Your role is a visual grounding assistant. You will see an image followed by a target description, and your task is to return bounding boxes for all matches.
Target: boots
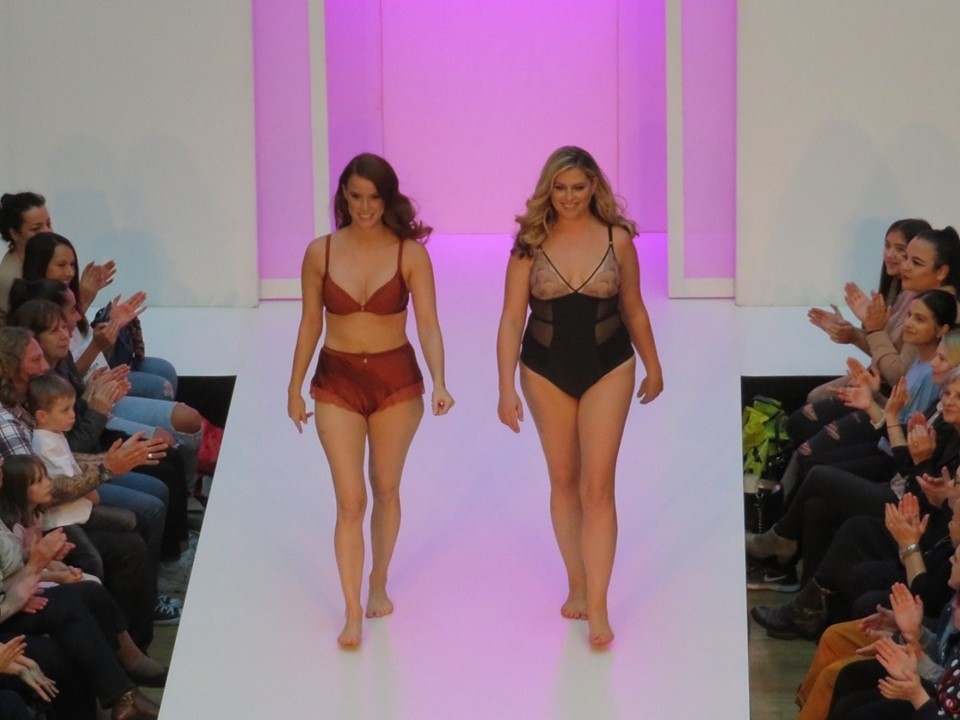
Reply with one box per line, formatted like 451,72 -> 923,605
750,578 -> 837,642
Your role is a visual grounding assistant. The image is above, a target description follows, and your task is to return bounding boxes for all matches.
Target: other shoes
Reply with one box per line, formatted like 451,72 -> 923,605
157,593 -> 183,608
153,600 -> 183,625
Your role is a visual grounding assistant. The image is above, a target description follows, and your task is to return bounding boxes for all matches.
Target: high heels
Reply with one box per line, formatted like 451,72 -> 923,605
745,524 -> 796,566
112,688 -> 159,719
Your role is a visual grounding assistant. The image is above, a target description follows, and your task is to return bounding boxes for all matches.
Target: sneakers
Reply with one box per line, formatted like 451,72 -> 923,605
164,556 -> 194,581
746,565 -> 801,592
180,545 -> 196,564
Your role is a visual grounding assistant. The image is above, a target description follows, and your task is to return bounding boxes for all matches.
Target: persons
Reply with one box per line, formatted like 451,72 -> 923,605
745,218 -> 960,719
496,147 -> 664,646
0,190 -> 211,720
287,152 -> 454,648
